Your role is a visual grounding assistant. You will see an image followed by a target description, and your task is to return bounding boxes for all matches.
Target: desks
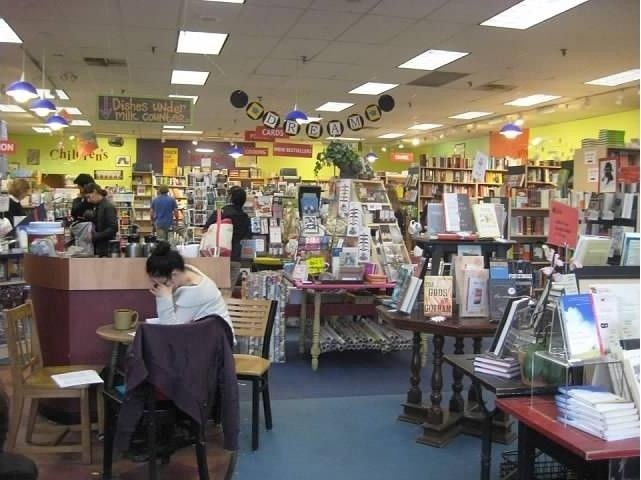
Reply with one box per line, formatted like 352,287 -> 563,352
284,276 -> 429,372
441,349 -> 570,480
494,397 -> 639,479
96,319 -> 146,389
19,250 -> 232,427
368,302 -> 519,450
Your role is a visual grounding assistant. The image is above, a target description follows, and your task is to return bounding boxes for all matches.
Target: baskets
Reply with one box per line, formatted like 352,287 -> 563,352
500,448 -> 584,480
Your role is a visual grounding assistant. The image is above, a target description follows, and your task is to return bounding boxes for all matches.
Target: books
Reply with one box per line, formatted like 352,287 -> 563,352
0,178 -> 52,225
52,195 -> 133,249
401,279 -> 423,315
153,177 -> 176,238
411,154 -> 559,321
396,275 -> 418,313
132,166 -> 151,240
50,368 -> 105,389
246,178 -> 409,311
176,166 -> 264,244
568,130 -> 638,232
476,232 -> 640,441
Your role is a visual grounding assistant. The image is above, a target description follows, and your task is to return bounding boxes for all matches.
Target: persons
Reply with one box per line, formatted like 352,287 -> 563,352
69,173 -> 98,221
150,184 -> 178,240
73,182 -> 120,259
82,240 -> 241,410
204,185 -> 253,290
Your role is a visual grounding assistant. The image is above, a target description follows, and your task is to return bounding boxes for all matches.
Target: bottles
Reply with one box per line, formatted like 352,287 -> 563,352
144,235 -> 160,257
127,234 -> 144,257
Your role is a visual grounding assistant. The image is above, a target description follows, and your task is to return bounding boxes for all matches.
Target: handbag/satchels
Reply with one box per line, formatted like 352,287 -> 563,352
200,208 -> 234,257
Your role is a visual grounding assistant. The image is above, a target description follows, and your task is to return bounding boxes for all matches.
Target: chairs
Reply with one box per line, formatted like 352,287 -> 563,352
0,297 -> 105,468
220,299 -> 278,453
99,310 -> 213,479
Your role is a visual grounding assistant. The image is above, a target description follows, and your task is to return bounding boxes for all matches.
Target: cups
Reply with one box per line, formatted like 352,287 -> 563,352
113,309 -> 139,329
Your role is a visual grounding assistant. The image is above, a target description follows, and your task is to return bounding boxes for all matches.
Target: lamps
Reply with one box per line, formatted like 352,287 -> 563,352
1,43 -> 41,106
43,92 -> 70,130
27,52 -> 58,117
495,122 -> 523,139
282,102 -> 309,125
